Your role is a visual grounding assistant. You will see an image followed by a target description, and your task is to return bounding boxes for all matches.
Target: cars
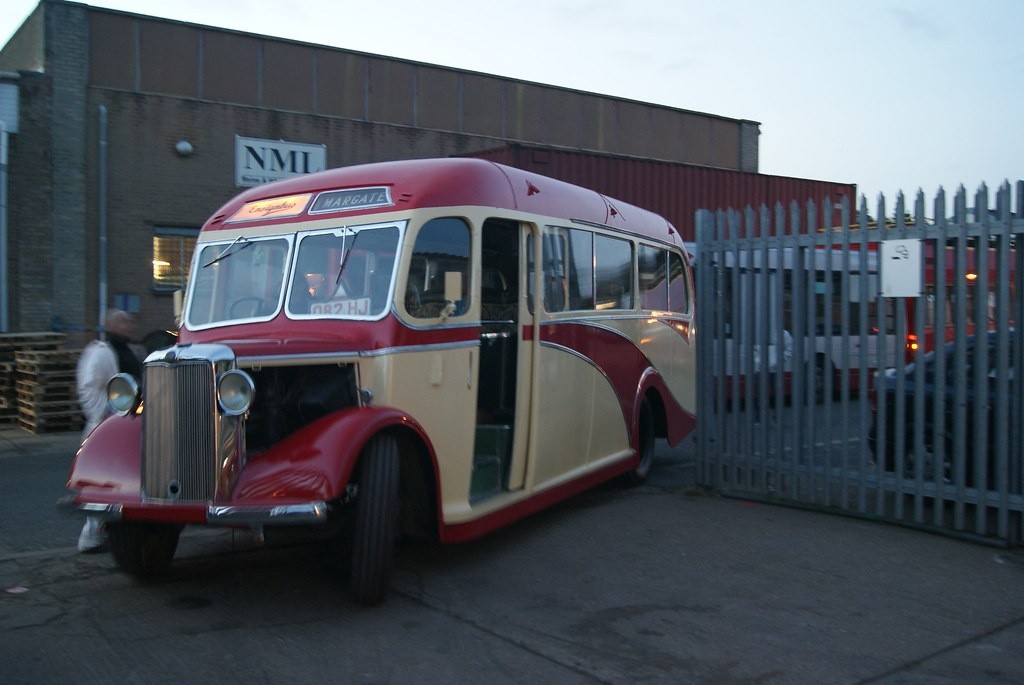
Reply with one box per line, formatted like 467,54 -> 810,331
867,326 -> 1023,517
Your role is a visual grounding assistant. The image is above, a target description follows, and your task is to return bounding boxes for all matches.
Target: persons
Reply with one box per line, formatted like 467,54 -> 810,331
76,309 -> 148,553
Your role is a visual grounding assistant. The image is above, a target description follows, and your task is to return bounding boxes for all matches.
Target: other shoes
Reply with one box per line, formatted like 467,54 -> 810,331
83,537 -> 114,554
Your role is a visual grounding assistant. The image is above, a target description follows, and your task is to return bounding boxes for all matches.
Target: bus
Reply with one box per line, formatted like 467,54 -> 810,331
673,241 -> 919,413
813,219 -> 1021,364
67,152 -> 697,611
